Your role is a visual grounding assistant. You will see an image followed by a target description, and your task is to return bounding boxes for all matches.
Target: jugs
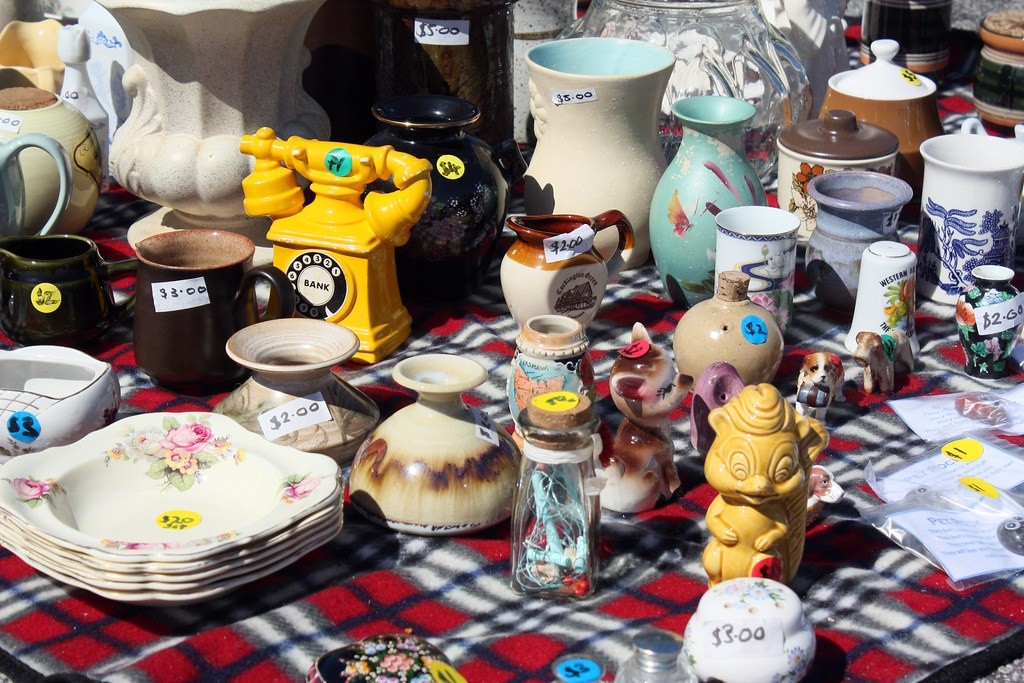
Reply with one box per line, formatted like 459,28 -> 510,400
134,230 -> 295,395
0,234 -> 137,351
500,210 -> 635,329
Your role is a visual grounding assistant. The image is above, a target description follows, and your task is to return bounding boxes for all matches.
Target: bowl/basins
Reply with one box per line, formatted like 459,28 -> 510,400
776,109 -> 899,239
0,346 -> 121,455
815,39 -> 945,226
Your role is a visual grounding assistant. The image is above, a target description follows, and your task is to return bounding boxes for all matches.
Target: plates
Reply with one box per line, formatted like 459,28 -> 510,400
0,412 -> 345,607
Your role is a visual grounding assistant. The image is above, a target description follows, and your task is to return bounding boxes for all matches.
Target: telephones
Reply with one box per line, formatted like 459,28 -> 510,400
238,124 -> 434,366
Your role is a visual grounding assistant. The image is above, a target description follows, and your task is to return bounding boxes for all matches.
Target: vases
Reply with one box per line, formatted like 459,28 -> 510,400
1,0 -> 848,307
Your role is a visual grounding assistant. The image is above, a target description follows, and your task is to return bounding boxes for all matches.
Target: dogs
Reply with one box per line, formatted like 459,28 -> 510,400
806,464 -> 846,510
853,331 -> 916,398
794,352 -> 847,427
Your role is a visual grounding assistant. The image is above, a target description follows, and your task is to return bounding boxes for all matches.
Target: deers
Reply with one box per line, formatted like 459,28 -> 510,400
595,321 -> 694,513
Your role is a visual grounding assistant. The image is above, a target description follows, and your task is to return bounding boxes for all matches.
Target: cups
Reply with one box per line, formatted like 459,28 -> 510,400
861,0 -> 953,79
916,134 -> 1024,305
0,133 -> 72,235
714,206 -> 800,334
845,241 -> 920,354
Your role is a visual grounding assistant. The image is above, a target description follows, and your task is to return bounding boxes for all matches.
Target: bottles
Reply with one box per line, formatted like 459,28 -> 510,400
972,9 -> 1024,126
508,391 -> 600,599
213,317 -> 379,467
524,38 -> 676,269
955,265 -> 1024,379
507,315 -> 596,453
361,94 -> 528,304
673,271 -> 784,386
613,630 -> 698,683
346,354 -> 522,534
649,96 -> 769,308
54,23 -> 112,193
